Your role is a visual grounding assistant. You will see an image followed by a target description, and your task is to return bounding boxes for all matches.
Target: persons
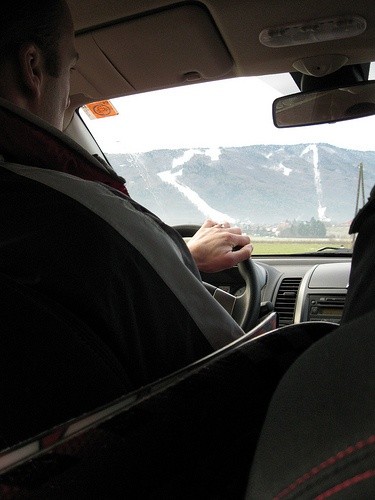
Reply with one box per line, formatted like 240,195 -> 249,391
0,0 -> 255,500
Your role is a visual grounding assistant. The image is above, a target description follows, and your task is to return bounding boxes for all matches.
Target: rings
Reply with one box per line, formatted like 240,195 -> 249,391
218,223 -> 225,228
